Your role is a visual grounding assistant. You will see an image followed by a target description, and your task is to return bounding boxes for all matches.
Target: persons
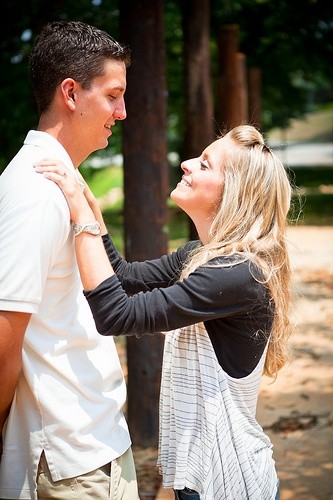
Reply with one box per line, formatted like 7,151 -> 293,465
0,21 -> 139,500
32,125 -> 304,500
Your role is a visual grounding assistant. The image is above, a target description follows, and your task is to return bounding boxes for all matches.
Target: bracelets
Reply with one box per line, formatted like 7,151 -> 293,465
72,221 -> 101,237
